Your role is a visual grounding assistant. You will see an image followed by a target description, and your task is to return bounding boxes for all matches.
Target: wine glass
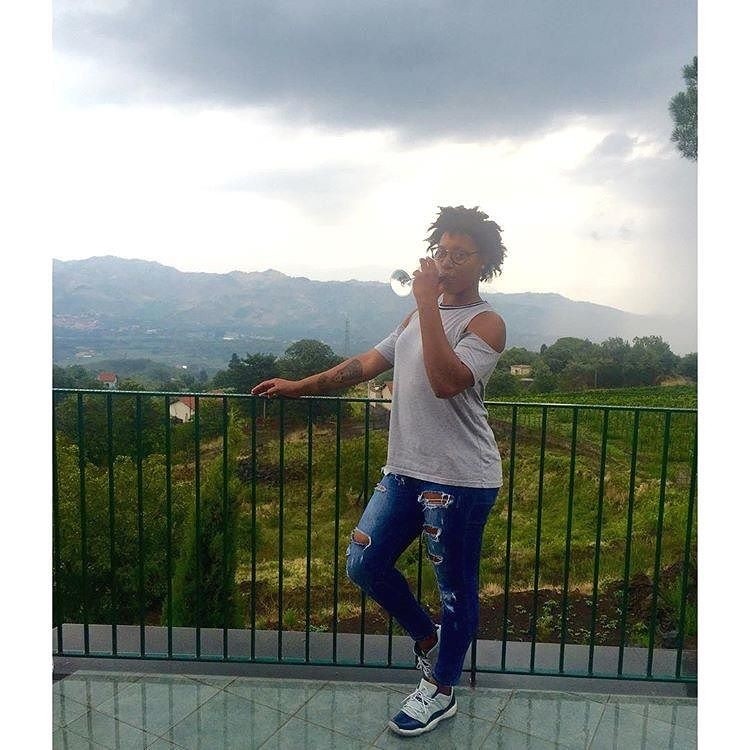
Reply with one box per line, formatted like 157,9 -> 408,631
390,260 -> 452,297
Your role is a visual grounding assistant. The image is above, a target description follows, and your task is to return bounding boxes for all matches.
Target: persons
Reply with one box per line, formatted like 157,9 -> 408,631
251,205 -> 506,736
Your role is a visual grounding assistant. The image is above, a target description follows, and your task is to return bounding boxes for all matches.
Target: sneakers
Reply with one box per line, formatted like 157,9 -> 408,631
387,679 -> 457,737
413,623 -> 442,678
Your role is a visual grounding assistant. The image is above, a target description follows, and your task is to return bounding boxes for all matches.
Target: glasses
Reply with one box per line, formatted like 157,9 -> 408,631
430,246 -> 481,266
